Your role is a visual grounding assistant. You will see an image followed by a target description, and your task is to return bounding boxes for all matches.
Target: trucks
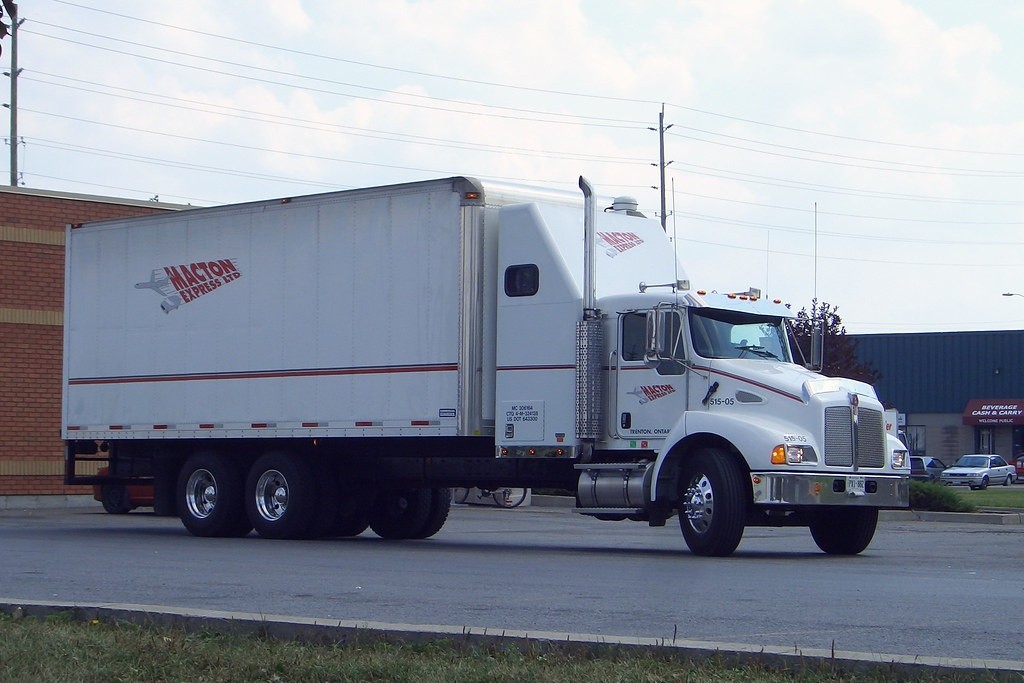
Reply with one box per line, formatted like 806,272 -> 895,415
882,407 -> 912,453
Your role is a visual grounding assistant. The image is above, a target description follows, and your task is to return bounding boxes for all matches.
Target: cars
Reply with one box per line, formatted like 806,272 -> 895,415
1008,453 -> 1024,484
939,454 -> 1016,491
910,455 -> 951,482
90,463 -> 156,515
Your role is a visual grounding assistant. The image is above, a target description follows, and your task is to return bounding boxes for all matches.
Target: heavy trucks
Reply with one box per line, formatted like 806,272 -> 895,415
61,173 -> 914,557
